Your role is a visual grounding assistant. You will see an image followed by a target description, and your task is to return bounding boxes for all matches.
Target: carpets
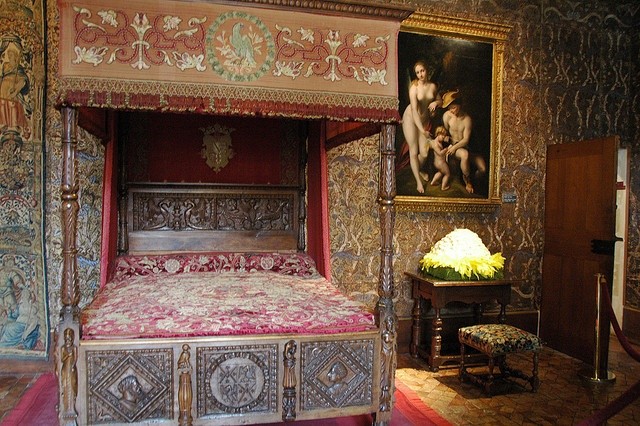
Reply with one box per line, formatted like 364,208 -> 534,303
1,370 -> 455,426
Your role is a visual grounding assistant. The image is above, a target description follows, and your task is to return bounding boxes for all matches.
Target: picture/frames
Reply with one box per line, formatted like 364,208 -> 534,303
394,10 -> 516,212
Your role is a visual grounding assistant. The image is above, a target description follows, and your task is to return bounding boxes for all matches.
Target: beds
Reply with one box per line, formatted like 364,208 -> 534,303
61,1 -> 419,423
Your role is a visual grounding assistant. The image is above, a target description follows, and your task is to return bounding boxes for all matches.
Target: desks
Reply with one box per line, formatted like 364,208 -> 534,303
403,269 -> 525,371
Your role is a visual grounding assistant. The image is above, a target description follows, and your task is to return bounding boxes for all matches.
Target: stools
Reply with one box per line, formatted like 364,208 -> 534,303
459,323 -> 546,395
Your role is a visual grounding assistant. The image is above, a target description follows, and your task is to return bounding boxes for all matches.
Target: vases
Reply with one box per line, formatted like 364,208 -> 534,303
421,265 -> 503,283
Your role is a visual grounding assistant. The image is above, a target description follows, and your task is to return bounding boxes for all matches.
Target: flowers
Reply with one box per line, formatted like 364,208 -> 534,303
419,228 -> 507,281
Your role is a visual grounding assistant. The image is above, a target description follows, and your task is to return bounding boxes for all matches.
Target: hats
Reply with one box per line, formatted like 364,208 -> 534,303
441,89 -> 463,109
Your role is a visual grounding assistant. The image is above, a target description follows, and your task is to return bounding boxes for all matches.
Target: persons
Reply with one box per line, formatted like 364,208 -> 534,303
442,91 -> 487,193
431,125 -> 451,190
402,62 -> 443,193
